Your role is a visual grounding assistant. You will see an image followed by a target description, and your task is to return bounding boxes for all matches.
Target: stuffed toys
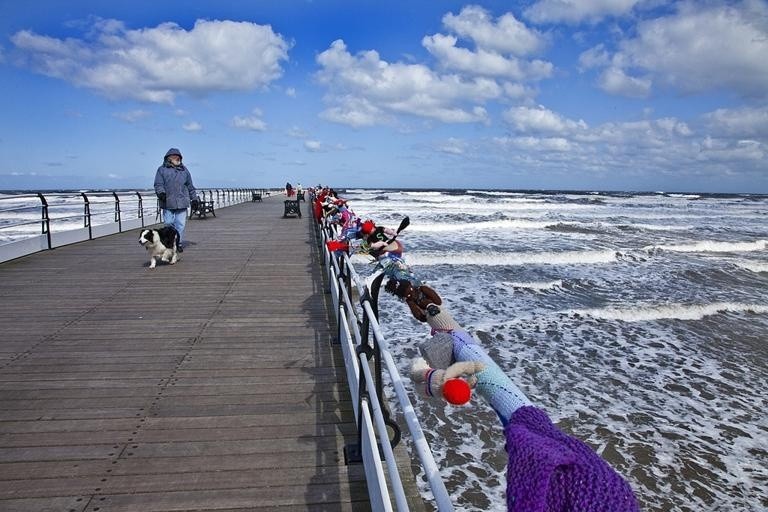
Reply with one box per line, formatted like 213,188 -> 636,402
307,185 -> 483,404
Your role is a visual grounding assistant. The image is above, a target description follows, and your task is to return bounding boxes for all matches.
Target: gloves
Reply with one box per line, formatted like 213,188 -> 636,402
191,200 -> 199,210
158,192 -> 166,202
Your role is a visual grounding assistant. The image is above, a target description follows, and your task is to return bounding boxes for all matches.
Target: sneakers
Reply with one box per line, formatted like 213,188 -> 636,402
176,245 -> 183,252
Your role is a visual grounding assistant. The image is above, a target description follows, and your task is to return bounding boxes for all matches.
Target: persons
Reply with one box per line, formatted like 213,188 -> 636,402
296,183 -> 302,195
154,148 -> 198,253
286,182 -> 292,197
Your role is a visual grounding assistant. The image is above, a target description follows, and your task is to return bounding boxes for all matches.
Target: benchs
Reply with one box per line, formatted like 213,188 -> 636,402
188,196 -> 217,219
251,192 -> 263,202
283,190 -> 306,218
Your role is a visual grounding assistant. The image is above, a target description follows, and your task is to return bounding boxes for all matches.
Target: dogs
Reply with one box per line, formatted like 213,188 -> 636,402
138,226 -> 183,270
